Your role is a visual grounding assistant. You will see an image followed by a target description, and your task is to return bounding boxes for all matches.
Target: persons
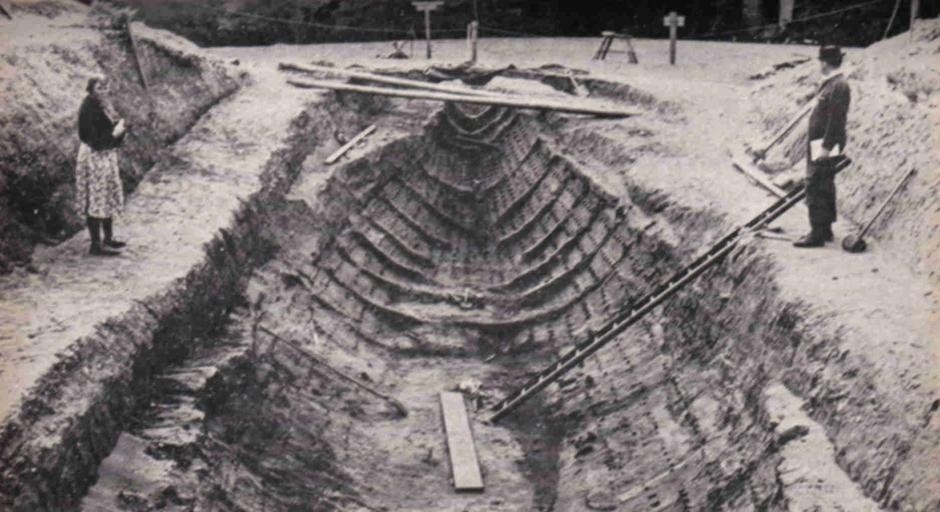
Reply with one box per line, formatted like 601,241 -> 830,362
792,44 -> 851,248
465,13 -> 479,65
74,73 -> 126,255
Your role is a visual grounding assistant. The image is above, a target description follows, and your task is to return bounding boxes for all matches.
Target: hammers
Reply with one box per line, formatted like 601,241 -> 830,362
842,166 -> 914,253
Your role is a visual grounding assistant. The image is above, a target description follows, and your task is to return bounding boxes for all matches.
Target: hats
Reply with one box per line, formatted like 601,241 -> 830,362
818,45 -> 846,64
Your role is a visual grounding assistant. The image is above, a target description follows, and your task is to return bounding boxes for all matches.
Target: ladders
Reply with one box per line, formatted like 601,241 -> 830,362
481,152 -> 853,425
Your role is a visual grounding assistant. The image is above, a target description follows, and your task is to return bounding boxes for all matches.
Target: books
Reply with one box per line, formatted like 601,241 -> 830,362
810,137 -> 839,161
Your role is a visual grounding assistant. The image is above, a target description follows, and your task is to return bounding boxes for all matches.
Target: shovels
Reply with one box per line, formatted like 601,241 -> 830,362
259,325 -> 407,417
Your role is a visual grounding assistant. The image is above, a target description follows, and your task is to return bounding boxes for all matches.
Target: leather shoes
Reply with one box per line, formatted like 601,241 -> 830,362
793,231 -> 825,248
104,238 -> 126,248
90,243 -> 121,255
